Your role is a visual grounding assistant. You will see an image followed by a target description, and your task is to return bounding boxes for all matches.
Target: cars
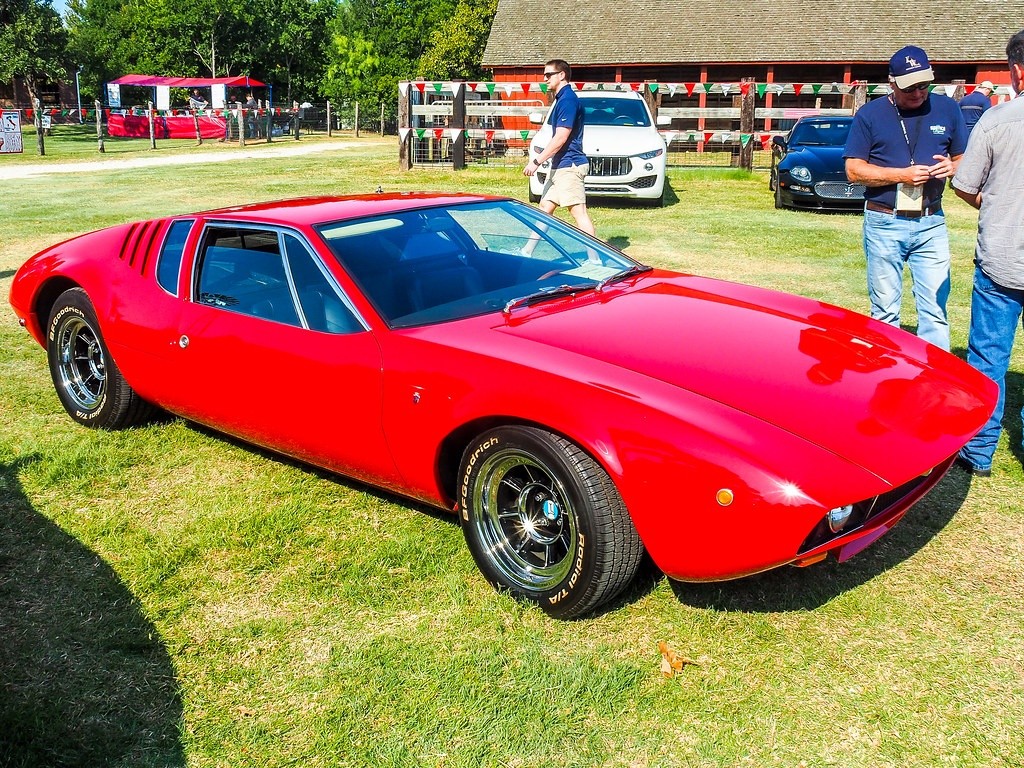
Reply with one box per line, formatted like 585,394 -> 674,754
8,188 -> 1000,620
771,115 -> 874,211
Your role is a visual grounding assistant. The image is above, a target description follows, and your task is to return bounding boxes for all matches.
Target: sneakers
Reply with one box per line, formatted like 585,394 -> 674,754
580,258 -> 603,266
500,247 -> 523,256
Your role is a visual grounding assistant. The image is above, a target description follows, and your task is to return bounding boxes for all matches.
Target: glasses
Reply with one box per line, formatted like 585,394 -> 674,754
544,71 -> 560,78
895,82 -> 930,93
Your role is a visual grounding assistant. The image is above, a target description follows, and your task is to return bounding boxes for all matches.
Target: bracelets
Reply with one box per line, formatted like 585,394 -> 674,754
533,159 -> 540,166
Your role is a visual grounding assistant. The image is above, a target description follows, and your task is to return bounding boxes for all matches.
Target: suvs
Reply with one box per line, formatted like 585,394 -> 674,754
528,89 -> 672,205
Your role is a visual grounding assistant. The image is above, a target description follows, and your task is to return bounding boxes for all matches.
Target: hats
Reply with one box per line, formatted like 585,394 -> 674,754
889,45 -> 934,90
978,81 -> 995,92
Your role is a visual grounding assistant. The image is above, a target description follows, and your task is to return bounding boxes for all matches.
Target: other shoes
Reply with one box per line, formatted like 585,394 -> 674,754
956,455 -> 991,478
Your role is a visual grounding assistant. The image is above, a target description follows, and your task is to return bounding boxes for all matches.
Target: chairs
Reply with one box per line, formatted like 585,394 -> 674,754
397,264 -> 486,315
799,126 -> 821,142
248,288 -> 353,332
608,104 -> 638,123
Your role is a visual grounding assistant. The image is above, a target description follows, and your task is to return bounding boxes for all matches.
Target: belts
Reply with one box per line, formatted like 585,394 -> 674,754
866,200 -> 941,217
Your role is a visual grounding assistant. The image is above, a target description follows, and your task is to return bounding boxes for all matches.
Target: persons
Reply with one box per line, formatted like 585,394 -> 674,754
229,96 -> 236,104
958,80 -> 995,134
841,45 -> 969,353
950,28 -> 1024,478
245,94 -> 258,139
498,60 -> 602,266
186,88 -> 208,108
